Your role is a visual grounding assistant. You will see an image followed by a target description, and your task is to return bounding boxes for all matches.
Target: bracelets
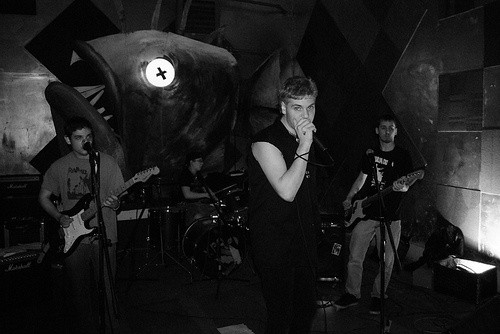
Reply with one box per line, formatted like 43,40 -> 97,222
294,152 -> 309,161
114,200 -> 120,210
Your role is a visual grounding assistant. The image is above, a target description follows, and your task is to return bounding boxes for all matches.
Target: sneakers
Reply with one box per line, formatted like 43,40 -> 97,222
368,297 -> 382,315
334,293 -> 360,309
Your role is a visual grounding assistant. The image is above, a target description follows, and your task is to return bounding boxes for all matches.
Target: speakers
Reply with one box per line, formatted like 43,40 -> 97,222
0,197 -> 60,250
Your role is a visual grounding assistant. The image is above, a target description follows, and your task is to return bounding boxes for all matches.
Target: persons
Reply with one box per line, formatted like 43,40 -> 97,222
246,77 -> 322,334
39,116 -> 128,334
179,152 -> 218,222
333,114 -> 414,314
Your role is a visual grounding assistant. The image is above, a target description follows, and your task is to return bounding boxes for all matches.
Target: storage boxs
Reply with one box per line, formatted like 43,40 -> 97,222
432,256 -> 498,305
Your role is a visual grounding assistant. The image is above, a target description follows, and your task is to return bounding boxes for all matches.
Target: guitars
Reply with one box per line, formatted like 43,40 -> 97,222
47,165 -> 160,257
344,169 -> 425,226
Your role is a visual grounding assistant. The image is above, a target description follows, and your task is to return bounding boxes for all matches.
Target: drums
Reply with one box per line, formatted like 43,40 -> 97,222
224,187 -> 248,218
147,205 -> 186,251
182,215 -> 241,271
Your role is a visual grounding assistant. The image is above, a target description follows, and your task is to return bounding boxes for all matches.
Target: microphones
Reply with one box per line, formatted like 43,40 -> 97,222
83,142 -> 97,157
313,133 -> 327,151
365,148 -> 376,162
